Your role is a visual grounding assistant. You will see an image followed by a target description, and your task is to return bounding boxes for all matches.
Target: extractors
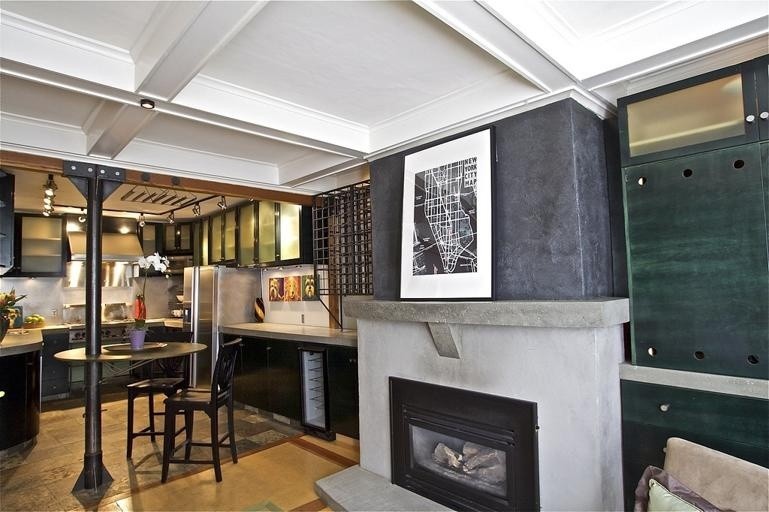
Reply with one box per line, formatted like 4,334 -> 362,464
60,212 -> 145,289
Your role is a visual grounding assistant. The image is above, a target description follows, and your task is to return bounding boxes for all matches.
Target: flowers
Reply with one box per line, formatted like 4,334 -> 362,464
0,289 -> 29,322
129,252 -> 170,328
7,285 -> 22,319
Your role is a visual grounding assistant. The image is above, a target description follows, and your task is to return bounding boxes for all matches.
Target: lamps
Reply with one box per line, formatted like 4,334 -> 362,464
139,212 -> 146,226
43,172 -> 57,219
192,201 -> 201,216
167,209 -> 175,224
217,195 -> 228,211
78,215 -> 89,224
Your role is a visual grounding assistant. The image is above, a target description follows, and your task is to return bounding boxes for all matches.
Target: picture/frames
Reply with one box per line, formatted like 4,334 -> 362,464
396,124 -> 498,303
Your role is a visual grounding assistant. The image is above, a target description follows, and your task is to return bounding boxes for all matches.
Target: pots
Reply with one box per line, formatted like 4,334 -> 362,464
104,303 -> 129,321
62,303 -> 86,324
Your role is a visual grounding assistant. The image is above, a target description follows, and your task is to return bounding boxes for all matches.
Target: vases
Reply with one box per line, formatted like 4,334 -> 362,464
9,321 -> 14,329
0,321 -> 10,343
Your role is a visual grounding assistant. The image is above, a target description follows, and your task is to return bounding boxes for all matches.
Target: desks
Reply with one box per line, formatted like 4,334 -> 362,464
55,340 -> 206,462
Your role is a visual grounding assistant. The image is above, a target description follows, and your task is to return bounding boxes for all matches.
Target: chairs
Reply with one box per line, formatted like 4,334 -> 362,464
126,331 -> 194,460
160,339 -> 243,484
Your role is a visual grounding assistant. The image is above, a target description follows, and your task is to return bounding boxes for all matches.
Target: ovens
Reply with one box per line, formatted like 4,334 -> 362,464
391,377 -> 541,512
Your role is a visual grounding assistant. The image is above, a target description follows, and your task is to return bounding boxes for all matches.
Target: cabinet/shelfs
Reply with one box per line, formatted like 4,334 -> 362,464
301,340 -> 336,441
277,203 -> 313,265
209,206 -> 236,266
163,221 -> 194,257
219,333 -> 301,420
137,222 -> 164,257
199,215 -> 209,266
14,212 -> 68,279
40,330 -> 72,403
133,327 -> 194,386
236,200 -> 281,269
617,54 -> 769,168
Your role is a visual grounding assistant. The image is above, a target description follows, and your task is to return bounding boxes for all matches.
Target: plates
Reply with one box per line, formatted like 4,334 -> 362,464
103,342 -> 168,354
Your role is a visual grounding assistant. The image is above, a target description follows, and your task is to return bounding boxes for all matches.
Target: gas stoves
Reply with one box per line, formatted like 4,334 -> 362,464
69,325 -> 129,343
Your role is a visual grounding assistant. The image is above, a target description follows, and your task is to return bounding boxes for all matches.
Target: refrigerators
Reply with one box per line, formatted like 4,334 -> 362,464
182,264 -> 263,393
